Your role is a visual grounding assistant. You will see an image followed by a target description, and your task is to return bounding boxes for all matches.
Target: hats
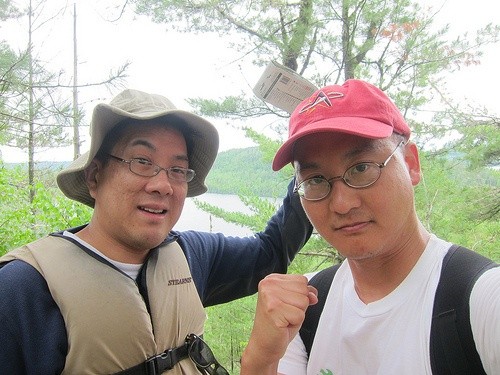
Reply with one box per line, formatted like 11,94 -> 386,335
56,89 -> 219,208
272,79 -> 411,172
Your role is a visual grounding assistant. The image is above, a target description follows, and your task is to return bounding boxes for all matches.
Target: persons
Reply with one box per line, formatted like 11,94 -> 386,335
239,79 -> 500,375
0,89 -> 314,375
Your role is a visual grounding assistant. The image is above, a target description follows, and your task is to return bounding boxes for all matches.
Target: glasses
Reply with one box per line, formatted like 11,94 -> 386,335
293,141 -> 405,201
185,333 -> 230,375
103,152 -> 196,182
